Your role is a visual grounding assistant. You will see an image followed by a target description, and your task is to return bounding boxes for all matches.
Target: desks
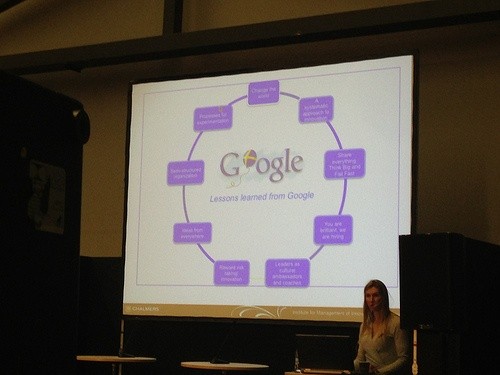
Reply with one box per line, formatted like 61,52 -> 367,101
180,362 -> 269,375
284,369 -> 352,375
76,355 -> 157,375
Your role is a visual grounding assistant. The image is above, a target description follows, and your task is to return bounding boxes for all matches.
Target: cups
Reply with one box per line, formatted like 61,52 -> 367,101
359,361 -> 371,375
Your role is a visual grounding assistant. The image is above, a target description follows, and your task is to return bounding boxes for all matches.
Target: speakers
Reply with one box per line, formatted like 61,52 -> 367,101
399,232 -> 500,375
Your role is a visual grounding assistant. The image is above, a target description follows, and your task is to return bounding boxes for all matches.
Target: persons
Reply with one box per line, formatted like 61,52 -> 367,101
354,280 -> 411,375
